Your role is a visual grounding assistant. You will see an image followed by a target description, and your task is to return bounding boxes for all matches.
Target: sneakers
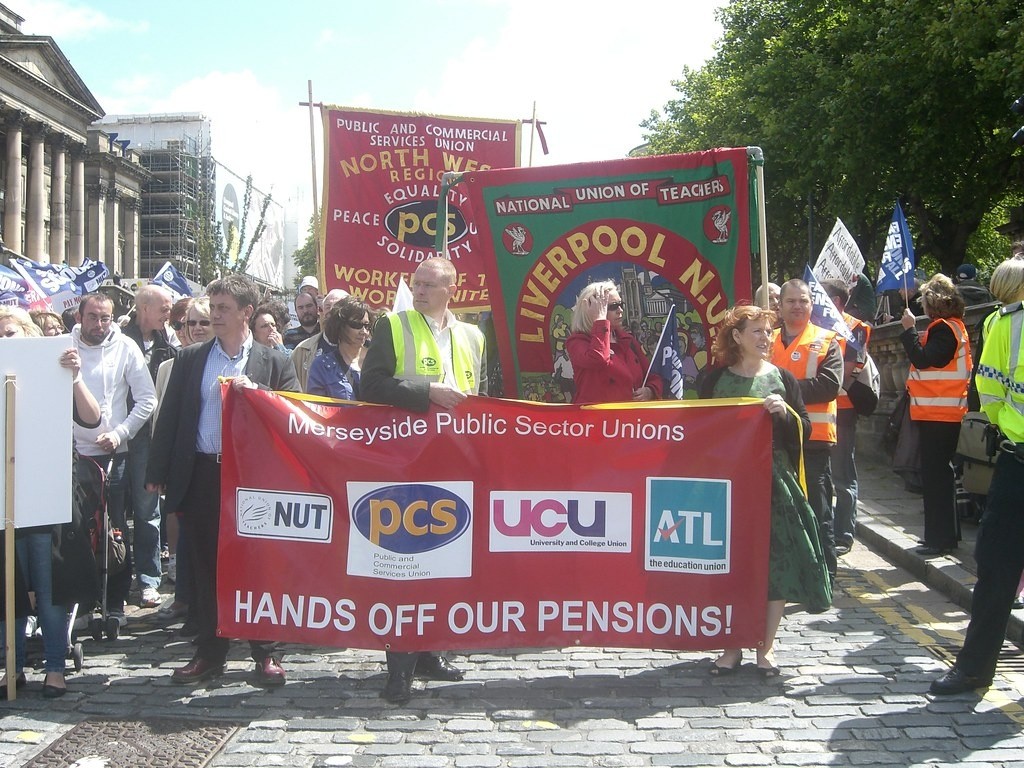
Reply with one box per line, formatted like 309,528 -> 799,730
69,548 -> 201,647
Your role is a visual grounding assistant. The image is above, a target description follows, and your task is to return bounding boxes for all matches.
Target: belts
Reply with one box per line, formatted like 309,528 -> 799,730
195,453 -> 222,464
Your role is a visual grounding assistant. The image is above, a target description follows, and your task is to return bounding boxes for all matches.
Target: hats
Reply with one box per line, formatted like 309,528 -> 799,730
299,276 -> 319,293
957,263 -> 976,280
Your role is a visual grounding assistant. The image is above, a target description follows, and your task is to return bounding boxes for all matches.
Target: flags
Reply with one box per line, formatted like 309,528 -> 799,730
876,205 -> 915,293
650,306 -> 684,400
0,257 -> 194,311
805,264 -> 861,353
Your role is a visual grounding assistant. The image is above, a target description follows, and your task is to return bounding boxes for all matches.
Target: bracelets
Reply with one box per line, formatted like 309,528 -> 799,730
74,372 -> 82,384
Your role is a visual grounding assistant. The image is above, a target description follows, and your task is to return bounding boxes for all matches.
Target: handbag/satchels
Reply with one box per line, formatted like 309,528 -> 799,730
955,411 -> 1000,496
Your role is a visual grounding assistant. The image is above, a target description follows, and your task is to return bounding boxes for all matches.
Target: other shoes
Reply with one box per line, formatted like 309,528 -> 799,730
44,674 -> 67,697
24,615 -> 42,638
0,672 -> 26,699
1011,596 -> 1024,609
916,535 -> 960,556
834,542 -> 851,556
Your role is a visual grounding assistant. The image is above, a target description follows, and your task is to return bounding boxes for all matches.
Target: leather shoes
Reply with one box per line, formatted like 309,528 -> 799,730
172,656 -> 228,683
414,656 -> 466,681
256,656 -> 287,687
386,671 -> 414,703
929,666 -> 993,695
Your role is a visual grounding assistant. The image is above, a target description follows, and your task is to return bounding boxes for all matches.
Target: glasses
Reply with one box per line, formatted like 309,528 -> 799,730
346,320 -> 372,330
170,320 -> 186,331
607,301 -> 623,311
187,320 -> 210,326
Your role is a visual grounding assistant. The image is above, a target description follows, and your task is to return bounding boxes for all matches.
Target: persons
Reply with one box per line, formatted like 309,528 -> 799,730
565,282 -> 662,402
698,310 -> 812,678
902,276 -> 971,553
930,303 -> 1024,693
360,256 -> 489,702
968,258 -> 1024,608
144,275 -> 301,684
758,273 -> 923,553
0,274 -> 215,697
253,276 -> 368,400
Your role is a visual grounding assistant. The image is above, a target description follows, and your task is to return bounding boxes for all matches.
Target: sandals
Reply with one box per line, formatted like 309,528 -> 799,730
757,666 -> 780,678
712,650 -> 743,676
